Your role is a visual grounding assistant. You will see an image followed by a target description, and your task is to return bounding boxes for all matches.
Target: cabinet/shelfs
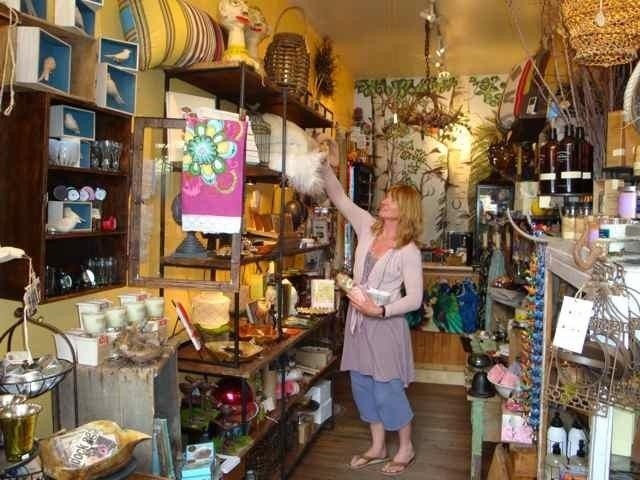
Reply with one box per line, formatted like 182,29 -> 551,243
542,238 -> 637,479
483,111 -> 595,480
157,59 -> 341,479
58,344 -> 182,472
0,89 -> 134,305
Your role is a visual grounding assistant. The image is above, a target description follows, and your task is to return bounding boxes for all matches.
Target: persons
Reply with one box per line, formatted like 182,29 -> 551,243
248,6 -> 270,66
316,141 -> 423,474
216,0 -> 250,55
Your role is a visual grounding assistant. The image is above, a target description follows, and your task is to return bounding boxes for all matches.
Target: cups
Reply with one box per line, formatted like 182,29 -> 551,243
146,297 -> 165,318
105,306 -> 126,328
81,314 -> 106,335
125,302 -> 145,321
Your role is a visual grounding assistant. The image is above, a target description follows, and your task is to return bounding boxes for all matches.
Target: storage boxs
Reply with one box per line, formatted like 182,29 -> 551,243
0,4 -> 98,105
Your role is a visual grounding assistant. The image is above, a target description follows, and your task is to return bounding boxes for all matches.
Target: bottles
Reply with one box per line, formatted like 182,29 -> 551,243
555,123 -> 577,193
617,186 -> 636,219
544,127 -> 558,192
539,130 -> 549,192
575,127 -> 594,192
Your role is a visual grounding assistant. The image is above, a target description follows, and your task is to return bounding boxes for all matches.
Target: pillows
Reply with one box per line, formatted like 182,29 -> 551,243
119,0 -> 225,70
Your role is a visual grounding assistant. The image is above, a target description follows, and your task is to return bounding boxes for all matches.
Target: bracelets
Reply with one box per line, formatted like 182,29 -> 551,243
379,304 -> 386,319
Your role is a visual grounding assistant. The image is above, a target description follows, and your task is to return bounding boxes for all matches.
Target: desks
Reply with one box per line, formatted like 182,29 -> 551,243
405,259 -> 482,387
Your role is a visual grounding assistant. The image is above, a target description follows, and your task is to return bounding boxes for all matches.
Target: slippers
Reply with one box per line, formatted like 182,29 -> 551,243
379,456 -> 417,476
349,453 -> 390,470
